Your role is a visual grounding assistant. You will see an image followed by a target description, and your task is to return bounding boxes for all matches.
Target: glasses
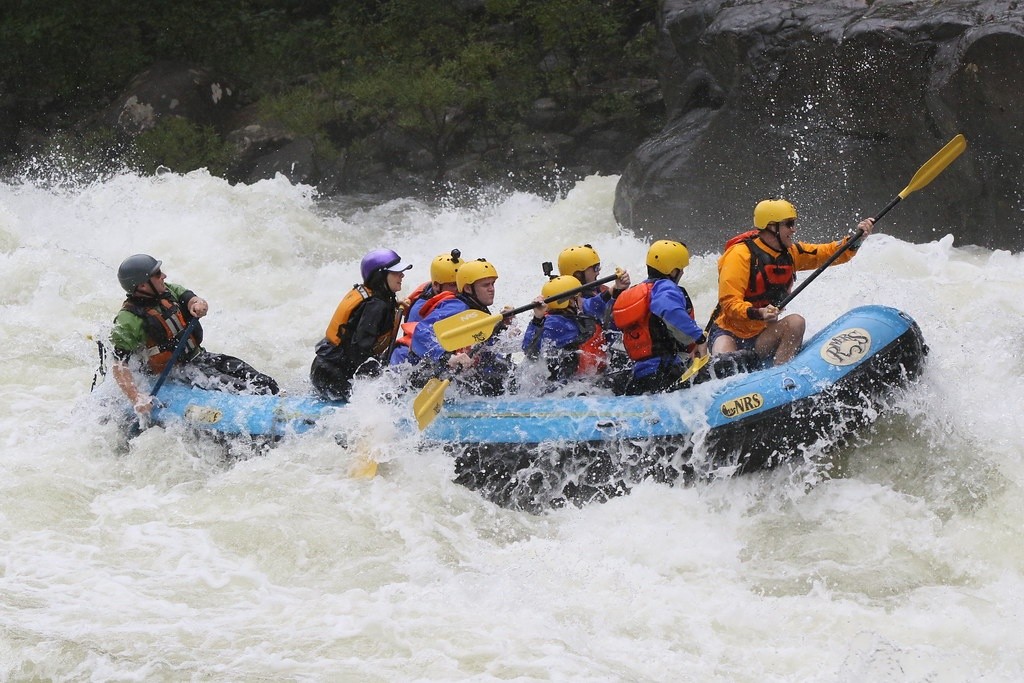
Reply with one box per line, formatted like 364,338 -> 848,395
768,219 -> 794,228
150,270 -> 162,278
590,264 -> 599,271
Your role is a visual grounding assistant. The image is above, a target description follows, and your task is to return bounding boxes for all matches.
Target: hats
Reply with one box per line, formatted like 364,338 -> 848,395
379,261 -> 413,271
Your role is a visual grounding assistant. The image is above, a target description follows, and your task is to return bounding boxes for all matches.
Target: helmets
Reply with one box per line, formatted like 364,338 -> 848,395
456,257 -> 498,292
753,197 -> 798,229
431,249 -> 466,285
360,248 -> 401,281
118,254 -> 162,293
646,239 -> 689,274
558,244 -> 599,275
541,262 -> 584,309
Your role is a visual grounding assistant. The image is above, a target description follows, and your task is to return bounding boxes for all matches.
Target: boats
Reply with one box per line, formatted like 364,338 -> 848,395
133,305 -> 930,513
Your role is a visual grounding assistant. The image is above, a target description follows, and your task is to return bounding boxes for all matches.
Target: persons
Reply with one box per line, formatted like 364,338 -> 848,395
524,240 -> 707,394
312,249 -> 413,401
708,200 -> 874,378
110,253 -> 279,415
390,240 -> 546,403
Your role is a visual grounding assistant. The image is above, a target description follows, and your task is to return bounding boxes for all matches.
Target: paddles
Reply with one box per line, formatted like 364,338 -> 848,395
124,308 -> 200,443
432,263 -> 628,351
679,301 -> 722,383
411,305 -> 512,430
769,131 -> 969,316
357,297 -> 408,486
